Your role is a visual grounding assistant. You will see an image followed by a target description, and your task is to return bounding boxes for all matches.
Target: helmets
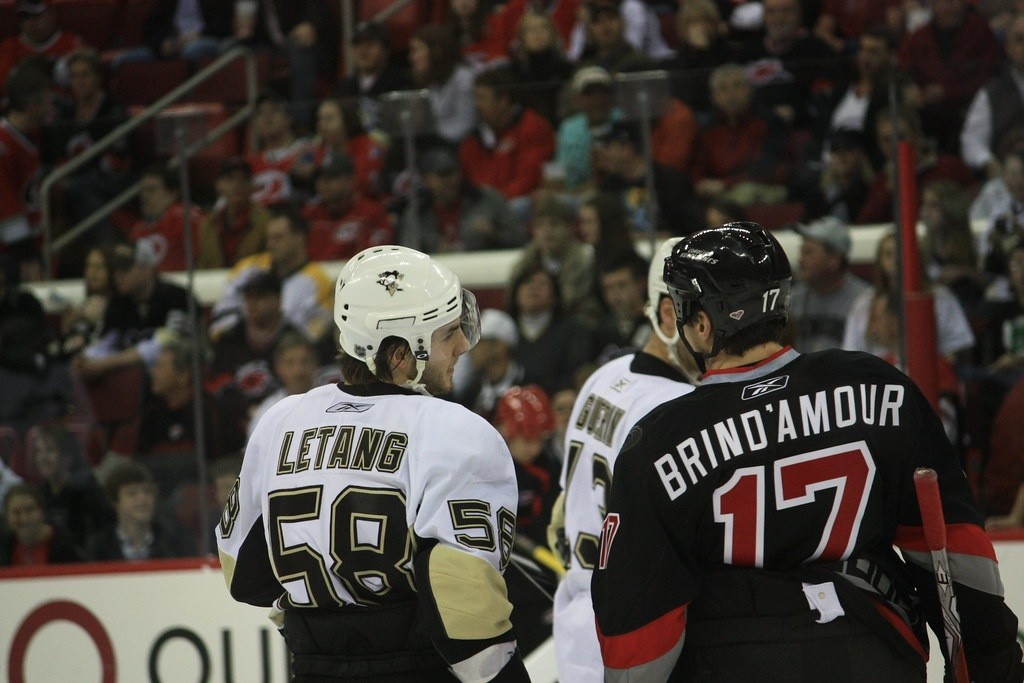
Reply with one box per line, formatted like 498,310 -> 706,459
498,385 -> 554,440
645,234 -> 689,314
668,221 -> 792,338
334,244 -> 463,373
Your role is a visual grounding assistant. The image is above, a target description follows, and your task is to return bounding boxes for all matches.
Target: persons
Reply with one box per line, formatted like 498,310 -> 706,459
3,2 -> 1024,567
590,220 -> 1023,682
214,245 -> 533,682
547,236 -> 703,682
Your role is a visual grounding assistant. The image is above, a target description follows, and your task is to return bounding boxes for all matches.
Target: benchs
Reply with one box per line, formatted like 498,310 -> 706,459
0,0 -> 420,248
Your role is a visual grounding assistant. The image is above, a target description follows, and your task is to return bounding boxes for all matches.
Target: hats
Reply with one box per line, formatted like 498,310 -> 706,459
793,217 -> 849,256
478,310 -> 517,347
572,68 -> 611,94
